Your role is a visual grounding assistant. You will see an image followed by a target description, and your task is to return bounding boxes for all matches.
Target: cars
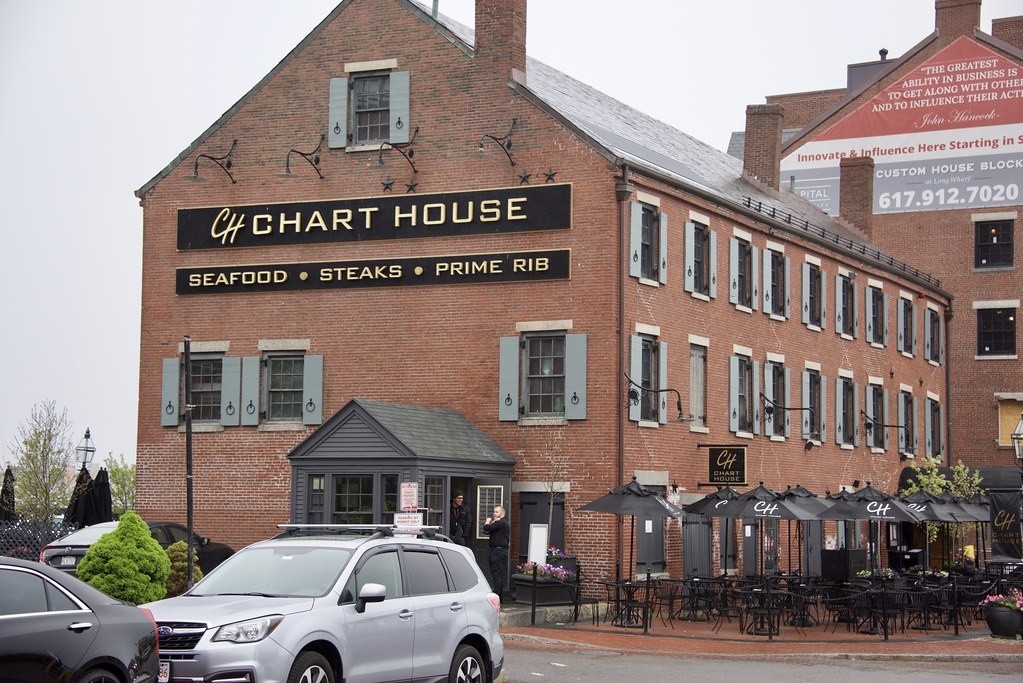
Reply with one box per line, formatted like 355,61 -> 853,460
40,521 -> 235,582
0,556 -> 160,683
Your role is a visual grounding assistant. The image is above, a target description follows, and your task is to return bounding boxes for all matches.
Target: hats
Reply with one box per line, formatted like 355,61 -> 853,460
451,488 -> 464,501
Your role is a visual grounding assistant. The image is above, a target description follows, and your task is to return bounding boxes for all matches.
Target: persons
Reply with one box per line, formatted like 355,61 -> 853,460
450,488 -> 472,545
483,506 -> 510,602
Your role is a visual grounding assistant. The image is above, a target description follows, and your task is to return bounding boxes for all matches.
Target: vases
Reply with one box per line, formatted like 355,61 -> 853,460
519,556 -> 576,572
985,607 -> 1023,636
512,574 -> 576,606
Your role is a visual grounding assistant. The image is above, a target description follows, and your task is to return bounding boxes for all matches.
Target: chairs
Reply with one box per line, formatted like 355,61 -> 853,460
567,570 -> 1023,638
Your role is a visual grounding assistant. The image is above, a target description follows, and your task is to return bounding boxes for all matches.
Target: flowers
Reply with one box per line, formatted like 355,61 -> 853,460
979,587 -> 1023,612
517,561 -> 576,583
546,546 -> 574,561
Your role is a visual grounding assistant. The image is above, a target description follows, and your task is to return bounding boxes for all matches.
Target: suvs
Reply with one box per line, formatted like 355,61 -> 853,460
137,524 -> 504,683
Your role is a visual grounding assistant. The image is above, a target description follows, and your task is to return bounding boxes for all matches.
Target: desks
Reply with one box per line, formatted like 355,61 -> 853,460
662,579 -> 718,622
770,575 -> 816,584
732,589 -> 785,635
594,582 -> 663,628
841,589 -> 902,635
781,586 -> 832,627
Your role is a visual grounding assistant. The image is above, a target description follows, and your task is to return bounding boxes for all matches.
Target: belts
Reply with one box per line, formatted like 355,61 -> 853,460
489,546 -> 503,549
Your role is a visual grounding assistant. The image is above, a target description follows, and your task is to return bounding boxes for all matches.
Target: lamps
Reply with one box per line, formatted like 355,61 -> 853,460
275,133 -> 326,179
759,392 -> 821,435
469,118 -> 518,166
624,372 -> 695,424
367,127 -> 419,173
860,410 -> 917,449
182,139 -> 238,184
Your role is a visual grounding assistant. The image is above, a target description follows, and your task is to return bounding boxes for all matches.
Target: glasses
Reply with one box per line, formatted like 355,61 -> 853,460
457,497 -> 464,500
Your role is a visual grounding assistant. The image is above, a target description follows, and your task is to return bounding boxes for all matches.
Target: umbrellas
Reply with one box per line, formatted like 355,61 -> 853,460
576,474 -> 687,628
681,481 -> 837,616
59,462 -> 106,535
94,467 -> 112,523
816,479 -> 1023,634
0,465 -> 16,526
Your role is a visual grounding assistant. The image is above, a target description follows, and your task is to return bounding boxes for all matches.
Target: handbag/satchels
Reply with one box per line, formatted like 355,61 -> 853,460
450,524 -> 463,542
